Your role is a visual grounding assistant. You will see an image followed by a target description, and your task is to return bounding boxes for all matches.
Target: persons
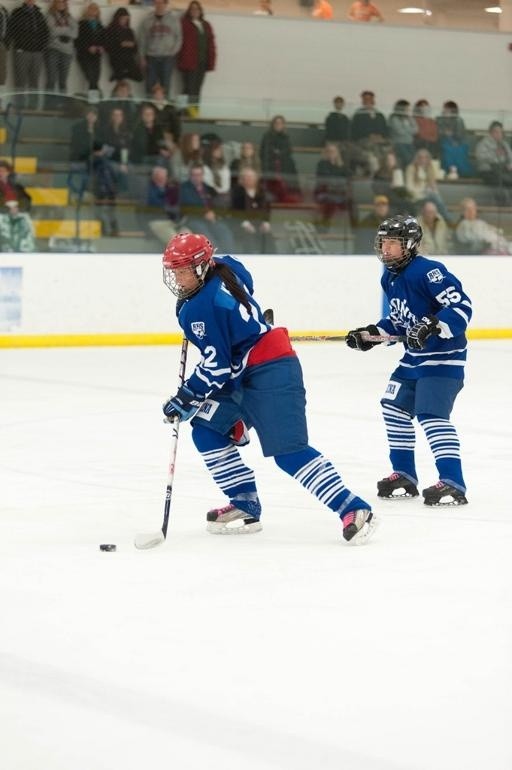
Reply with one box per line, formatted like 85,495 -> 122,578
1,160 -> 34,252
312,91 -> 511,253
254,2 -> 382,24
344,213 -> 472,498
260,115 -> 304,203
0,1 -> 216,113
69,82 -> 278,253
161,232 -> 372,541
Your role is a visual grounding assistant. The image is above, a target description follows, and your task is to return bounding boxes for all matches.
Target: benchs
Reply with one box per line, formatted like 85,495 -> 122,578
0,103 -> 512,255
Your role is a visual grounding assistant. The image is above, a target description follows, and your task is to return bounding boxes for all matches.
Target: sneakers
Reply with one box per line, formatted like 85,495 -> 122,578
343,508 -> 370,540
423,481 -> 464,497
377,473 -> 413,490
207,503 -> 254,522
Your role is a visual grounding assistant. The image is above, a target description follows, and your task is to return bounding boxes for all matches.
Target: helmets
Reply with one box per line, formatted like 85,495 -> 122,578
374,215 -> 422,271
163,233 -> 214,299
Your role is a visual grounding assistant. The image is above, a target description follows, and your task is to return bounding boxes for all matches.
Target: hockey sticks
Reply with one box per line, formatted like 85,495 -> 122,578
98,333 -> 189,552
264,309 -> 407,343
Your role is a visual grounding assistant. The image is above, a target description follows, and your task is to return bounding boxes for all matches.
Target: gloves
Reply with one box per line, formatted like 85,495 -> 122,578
345,324 -> 381,351
407,314 -> 441,351
164,381 -> 205,422
